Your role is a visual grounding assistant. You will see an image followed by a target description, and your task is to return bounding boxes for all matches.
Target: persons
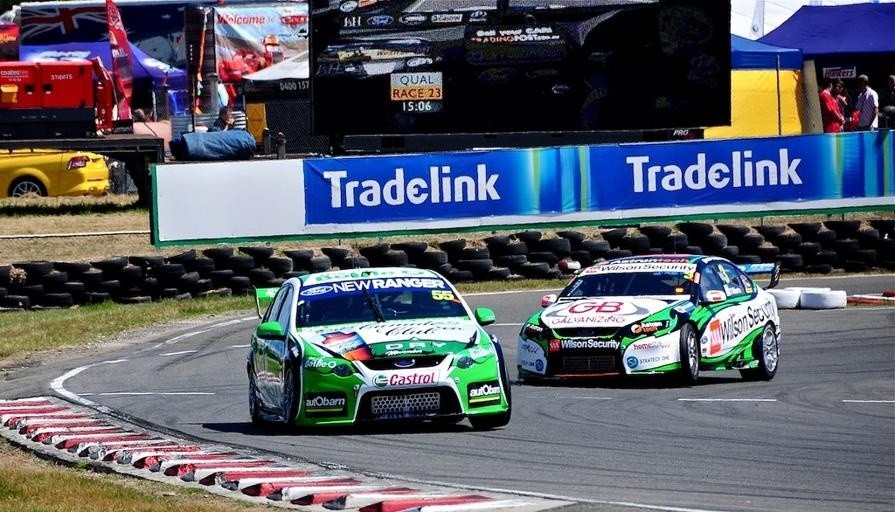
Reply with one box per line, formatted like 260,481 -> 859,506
819,75 -> 895,133
214,106 -> 235,131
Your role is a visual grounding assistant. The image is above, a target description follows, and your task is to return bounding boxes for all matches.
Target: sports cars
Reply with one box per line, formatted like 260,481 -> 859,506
517,254 -> 781,385
247,267 -> 511,430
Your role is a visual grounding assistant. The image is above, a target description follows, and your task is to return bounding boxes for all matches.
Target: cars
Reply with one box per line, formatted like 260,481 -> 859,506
0,148 -> 108,198
